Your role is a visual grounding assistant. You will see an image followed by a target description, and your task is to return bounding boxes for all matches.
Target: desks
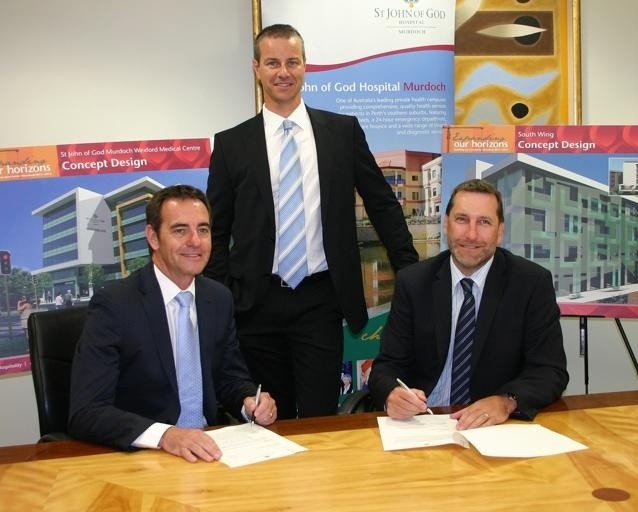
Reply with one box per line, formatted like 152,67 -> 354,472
0,393 -> 638,512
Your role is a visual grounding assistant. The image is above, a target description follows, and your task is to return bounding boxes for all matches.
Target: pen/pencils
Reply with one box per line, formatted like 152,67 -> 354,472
397,377 -> 433,416
251,384 -> 262,429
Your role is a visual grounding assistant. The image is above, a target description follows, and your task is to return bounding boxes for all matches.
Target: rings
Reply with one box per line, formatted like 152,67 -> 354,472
269,413 -> 274,418
484,413 -> 490,419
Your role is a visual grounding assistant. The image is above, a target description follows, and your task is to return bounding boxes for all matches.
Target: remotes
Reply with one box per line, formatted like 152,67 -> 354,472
568,293 -> 581,299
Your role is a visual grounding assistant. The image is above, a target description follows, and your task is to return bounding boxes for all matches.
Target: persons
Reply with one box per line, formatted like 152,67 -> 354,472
67,184 -> 277,463
17,295 -> 35,352
55,291 -> 63,309
367,179 -> 570,431
202,24 -> 420,419
64,289 -> 74,307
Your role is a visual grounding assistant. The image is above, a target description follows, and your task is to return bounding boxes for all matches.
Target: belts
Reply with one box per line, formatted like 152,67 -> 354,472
257,271 -> 330,286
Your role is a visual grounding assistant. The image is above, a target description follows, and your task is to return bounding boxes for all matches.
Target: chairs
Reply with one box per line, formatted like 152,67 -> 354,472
27,306 -> 94,442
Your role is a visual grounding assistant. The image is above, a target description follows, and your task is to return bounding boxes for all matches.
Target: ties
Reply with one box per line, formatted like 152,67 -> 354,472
448,280 -> 478,414
174,291 -> 205,434
276,121 -> 309,292
